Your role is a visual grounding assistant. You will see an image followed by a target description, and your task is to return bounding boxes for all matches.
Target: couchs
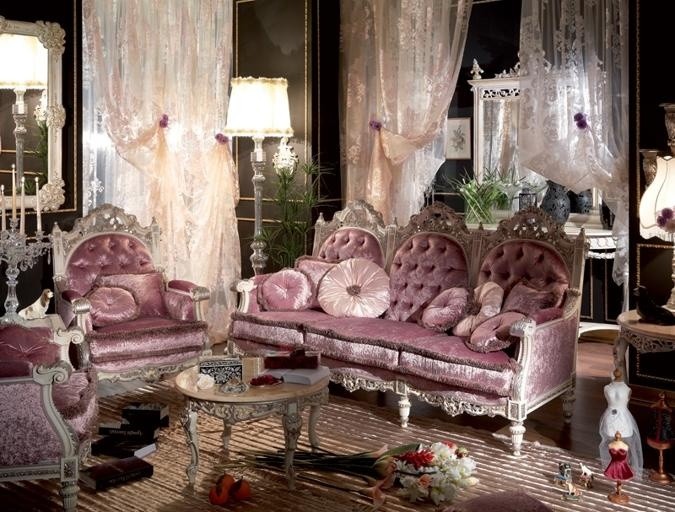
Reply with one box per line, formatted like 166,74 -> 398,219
225,198 -> 587,458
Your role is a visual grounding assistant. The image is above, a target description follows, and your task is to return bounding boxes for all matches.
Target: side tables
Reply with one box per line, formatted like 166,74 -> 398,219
613,309 -> 675,485
23,313 -> 85,372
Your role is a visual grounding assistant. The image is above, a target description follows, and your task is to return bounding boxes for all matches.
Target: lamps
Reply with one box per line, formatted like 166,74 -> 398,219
222,76 -> 294,275
0,33 -> 48,196
639,155 -> 675,312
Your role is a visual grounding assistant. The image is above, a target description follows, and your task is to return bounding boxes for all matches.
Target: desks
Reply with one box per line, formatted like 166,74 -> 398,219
465,223 -> 629,325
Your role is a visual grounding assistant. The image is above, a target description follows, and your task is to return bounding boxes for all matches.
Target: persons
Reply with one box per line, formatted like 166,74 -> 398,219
600,370 -> 635,439
648,392 -> 673,443
604,431 -> 634,483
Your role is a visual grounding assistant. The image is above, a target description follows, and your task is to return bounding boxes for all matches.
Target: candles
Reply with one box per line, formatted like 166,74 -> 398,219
0,165 -> 41,234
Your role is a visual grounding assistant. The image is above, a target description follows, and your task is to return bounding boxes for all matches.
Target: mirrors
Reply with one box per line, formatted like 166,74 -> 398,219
0,16 -> 66,213
467,50 -> 606,229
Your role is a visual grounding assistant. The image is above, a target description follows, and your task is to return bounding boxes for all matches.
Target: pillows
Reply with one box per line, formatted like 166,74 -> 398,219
97,270 -> 167,315
85,285 -> 139,328
0,324 -> 58,375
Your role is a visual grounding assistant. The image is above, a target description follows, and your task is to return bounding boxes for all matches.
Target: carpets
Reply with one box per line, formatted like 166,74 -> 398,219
0,376 -> 675,512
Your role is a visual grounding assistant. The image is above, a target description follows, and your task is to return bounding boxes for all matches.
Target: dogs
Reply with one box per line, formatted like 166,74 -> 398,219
17,288 -> 54,321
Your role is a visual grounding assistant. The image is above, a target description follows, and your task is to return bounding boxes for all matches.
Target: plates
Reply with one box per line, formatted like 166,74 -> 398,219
249,379 -> 283,388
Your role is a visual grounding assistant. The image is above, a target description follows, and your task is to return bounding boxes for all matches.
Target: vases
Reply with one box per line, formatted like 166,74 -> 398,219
540,179 -> 570,225
568,191 -> 592,213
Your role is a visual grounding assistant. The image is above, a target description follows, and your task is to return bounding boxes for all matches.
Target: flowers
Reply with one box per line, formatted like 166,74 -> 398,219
215,438 -> 479,508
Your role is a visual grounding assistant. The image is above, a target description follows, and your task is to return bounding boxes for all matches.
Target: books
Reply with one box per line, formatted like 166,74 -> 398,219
264,365 -> 330,386
264,351 -> 321,368
77,401 -> 169,491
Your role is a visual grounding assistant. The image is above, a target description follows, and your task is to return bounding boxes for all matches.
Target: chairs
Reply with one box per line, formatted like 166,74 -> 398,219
443,484 -> 553,512
49,203 -> 211,401
0,313 -> 99,512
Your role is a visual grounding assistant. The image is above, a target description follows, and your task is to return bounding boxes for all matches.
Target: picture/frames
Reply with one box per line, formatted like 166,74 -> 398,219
447,117 -> 471,160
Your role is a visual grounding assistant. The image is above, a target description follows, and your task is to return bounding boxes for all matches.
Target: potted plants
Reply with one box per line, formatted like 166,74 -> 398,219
442,168 -> 504,223
490,166 -> 526,210
